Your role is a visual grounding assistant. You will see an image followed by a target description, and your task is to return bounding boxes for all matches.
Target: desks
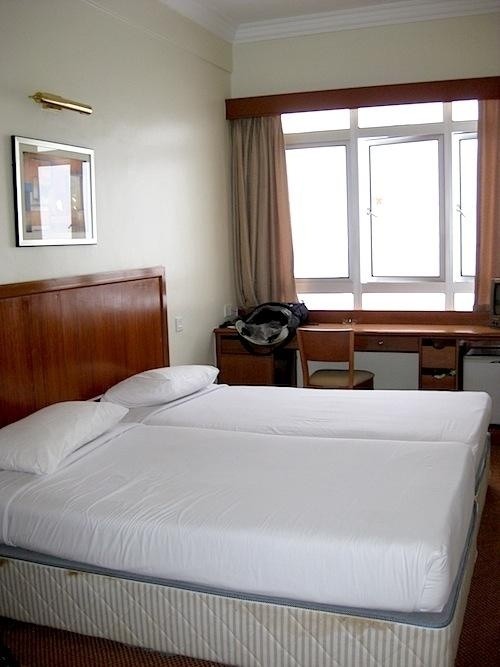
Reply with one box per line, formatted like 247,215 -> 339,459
213,310 -> 500,428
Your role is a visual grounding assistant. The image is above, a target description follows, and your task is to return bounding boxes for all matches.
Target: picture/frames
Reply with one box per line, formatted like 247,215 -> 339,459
11,135 -> 99,248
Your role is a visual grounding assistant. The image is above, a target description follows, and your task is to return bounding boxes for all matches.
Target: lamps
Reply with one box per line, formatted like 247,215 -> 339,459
35,93 -> 93,116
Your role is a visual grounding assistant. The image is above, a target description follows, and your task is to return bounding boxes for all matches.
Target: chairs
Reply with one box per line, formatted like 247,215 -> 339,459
293,327 -> 375,392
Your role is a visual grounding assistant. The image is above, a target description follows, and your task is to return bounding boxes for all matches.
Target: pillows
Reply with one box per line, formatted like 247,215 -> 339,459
101,364 -> 220,407
1,400 -> 129,477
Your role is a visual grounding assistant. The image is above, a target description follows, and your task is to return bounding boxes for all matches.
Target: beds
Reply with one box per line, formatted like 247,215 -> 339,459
1,267 -> 492,662
